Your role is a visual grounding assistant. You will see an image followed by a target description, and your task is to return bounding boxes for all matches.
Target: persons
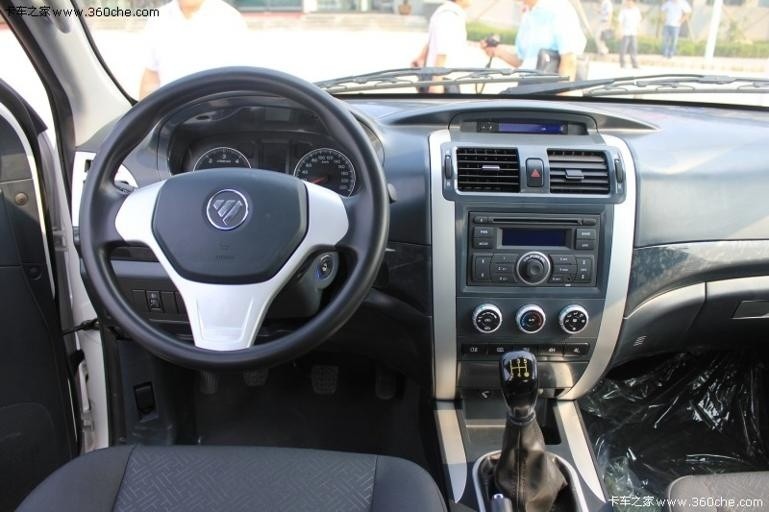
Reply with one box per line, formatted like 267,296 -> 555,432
660,0 -> 692,62
595,0 -> 614,63
411,0 -> 473,95
140,0 -> 255,101
617,0 -> 642,72
481,1 -> 587,98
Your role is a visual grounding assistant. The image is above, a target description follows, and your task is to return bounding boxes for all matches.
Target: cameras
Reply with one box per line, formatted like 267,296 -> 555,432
485,33 -> 500,47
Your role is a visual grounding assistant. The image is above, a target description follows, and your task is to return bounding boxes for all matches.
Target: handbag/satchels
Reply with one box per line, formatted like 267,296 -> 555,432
536,48 -> 589,82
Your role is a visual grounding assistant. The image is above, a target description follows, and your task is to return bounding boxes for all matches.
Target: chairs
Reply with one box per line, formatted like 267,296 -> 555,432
13,447 -> 769,511
